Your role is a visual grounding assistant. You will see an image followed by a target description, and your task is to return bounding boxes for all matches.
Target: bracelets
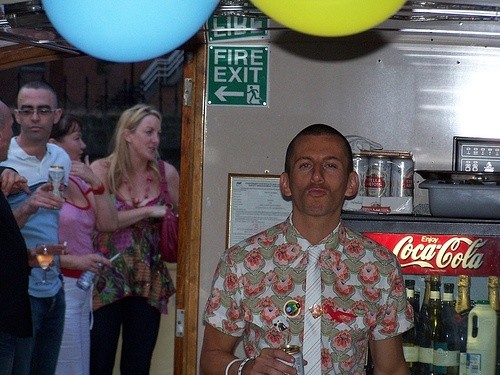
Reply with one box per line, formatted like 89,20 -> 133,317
238,357 -> 254,375
225,359 -> 244,375
93,183 -> 106,195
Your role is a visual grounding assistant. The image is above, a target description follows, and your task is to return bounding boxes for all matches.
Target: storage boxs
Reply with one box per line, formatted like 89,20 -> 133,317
418,179 -> 500,219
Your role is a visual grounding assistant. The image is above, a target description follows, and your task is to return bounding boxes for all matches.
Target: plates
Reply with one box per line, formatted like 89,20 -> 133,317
413,169 -> 500,182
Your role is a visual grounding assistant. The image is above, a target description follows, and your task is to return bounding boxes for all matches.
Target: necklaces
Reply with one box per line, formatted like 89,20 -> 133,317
124,175 -> 153,206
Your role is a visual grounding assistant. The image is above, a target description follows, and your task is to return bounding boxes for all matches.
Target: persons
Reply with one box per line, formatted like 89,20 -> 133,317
198,124 -> 414,375
48,113 -> 118,375
0,99 -> 66,375
90,103 -> 179,375
0,80 -> 72,375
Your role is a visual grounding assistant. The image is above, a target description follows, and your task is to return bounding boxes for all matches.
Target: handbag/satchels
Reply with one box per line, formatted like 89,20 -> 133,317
157,160 -> 178,264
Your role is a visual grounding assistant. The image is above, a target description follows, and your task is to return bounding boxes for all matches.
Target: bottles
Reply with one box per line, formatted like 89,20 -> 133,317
366,273 -> 500,375
76,245 -> 109,290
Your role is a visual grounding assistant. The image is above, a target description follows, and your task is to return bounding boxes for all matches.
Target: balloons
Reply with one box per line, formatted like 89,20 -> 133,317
41,0 -> 220,62
248,0 -> 407,37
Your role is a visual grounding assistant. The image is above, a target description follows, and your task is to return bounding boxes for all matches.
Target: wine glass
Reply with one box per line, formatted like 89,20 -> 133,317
35,243 -> 54,285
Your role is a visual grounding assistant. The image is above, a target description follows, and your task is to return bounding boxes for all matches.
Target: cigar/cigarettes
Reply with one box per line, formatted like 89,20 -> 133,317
61,241 -> 67,254
110,253 -> 120,261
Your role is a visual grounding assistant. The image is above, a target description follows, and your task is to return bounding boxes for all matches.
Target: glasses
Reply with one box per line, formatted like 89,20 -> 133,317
19,107 -> 56,117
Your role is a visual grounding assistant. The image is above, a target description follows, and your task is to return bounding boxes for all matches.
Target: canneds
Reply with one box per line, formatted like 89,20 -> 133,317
353,154 -> 368,196
276,345 -> 304,375
391,155 -> 414,197
47,165 -> 65,199
368,155 -> 390,197
